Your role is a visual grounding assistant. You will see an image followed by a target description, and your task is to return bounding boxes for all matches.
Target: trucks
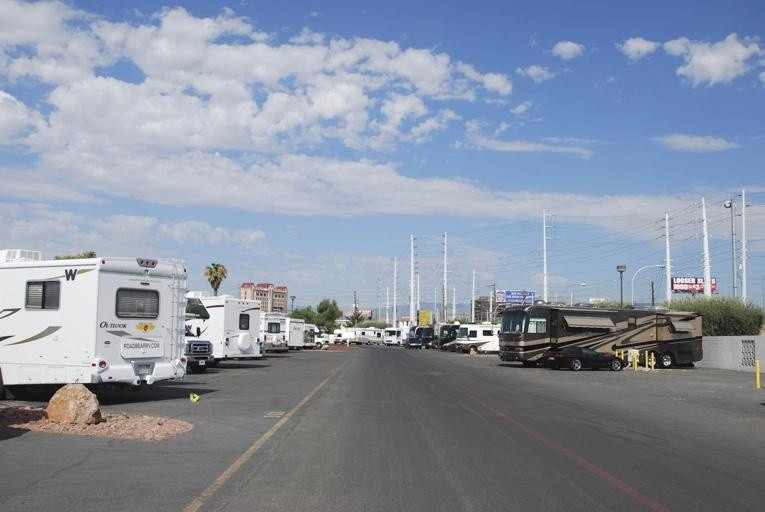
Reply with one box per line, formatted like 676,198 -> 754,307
0,249 -> 703,386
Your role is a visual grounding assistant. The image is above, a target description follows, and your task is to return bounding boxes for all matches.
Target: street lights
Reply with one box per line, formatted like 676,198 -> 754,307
723,199 -> 737,300
